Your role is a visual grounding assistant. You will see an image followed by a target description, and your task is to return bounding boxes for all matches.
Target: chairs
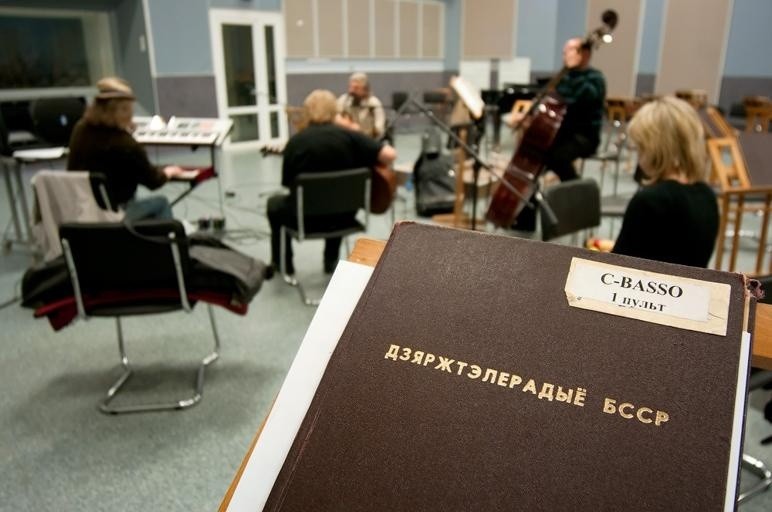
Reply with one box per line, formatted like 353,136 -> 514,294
746,96 -> 771,136
0,96 -> 85,245
59,216 -> 221,414
27,170 -> 115,254
691,101 -> 750,192
276,166 -> 371,308
289,105 -> 309,128
391,90 -> 411,118
604,95 -> 627,126
537,178 -> 600,249
716,183 -> 772,285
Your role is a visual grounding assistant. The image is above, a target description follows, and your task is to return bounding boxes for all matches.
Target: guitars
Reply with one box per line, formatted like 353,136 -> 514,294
262,146 -> 397,214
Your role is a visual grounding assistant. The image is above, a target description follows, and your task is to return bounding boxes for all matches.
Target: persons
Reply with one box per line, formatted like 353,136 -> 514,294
506,38 -> 606,232
266,89 -> 398,276
67,76 -> 183,224
333,72 -> 386,143
611,95 -> 719,268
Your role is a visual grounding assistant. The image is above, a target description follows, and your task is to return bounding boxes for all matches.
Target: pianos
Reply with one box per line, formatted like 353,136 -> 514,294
155,166 -> 213,186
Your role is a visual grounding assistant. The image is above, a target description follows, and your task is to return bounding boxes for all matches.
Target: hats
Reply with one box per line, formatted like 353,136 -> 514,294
95,77 -> 136,103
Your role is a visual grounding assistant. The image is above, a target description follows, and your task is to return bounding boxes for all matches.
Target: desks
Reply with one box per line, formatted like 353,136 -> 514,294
133,116 -> 234,222
750,301 -> 772,372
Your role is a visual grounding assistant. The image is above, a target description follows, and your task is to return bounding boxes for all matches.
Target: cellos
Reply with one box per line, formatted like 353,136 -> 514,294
486,10 -> 616,231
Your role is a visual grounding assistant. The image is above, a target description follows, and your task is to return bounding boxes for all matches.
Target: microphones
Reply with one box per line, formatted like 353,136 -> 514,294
534,191 -> 559,228
